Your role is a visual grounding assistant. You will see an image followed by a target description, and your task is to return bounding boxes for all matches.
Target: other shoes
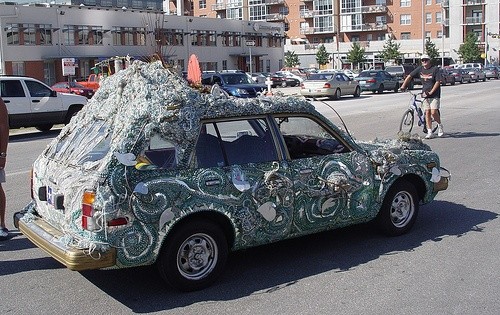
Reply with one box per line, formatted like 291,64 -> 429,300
438,126 -> 443,136
0,228 -> 9,241
425,133 -> 433,139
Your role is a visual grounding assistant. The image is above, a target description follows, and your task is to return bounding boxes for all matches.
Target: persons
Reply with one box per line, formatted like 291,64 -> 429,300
0,95 -> 17,240
399,54 -> 445,139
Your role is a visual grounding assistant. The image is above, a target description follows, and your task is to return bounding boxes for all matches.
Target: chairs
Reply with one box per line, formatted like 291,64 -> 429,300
195,134 -> 263,168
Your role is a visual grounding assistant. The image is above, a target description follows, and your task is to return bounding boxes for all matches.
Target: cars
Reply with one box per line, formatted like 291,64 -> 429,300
384,64 -> 423,90
300,73 -> 360,100
18,93 -> 449,286
243,71 -> 306,88
1,75 -> 87,134
281,69 -> 362,77
354,70 -> 399,94
437,67 -> 500,86
50,81 -> 94,100
203,73 -> 266,100
449,63 -> 484,69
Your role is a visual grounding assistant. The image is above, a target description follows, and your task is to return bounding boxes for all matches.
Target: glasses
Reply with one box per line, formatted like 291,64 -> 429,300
422,59 -> 429,62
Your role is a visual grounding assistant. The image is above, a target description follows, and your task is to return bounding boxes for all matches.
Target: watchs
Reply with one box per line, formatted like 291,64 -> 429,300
0,152 -> 6,159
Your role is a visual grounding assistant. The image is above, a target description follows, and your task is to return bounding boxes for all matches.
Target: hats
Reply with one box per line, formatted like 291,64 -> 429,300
421,54 -> 430,60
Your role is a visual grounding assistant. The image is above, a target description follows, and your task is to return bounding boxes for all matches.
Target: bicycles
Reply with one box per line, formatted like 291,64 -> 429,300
398,93 -> 442,135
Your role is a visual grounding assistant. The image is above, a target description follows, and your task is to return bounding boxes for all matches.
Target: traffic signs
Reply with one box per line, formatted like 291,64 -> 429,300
245,40 -> 257,46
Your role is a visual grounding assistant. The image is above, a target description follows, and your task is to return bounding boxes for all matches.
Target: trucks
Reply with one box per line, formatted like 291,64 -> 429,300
72,74 -> 101,93
